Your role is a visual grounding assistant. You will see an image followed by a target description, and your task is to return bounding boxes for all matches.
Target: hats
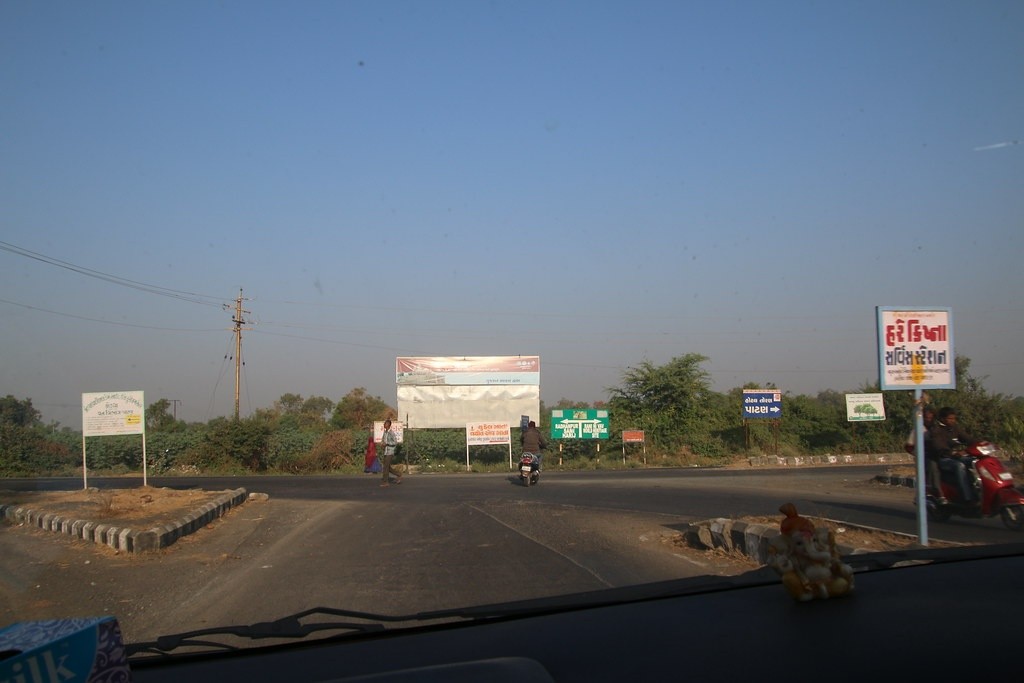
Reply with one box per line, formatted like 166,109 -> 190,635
528,421 -> 536,427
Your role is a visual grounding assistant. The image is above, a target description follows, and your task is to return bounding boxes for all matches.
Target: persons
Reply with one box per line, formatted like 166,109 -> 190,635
903,407 -> 978,508
379,420 -> 402,487
519,421 -> 545,458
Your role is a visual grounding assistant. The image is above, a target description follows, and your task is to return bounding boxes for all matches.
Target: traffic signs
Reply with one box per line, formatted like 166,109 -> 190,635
743,393 -> 782,418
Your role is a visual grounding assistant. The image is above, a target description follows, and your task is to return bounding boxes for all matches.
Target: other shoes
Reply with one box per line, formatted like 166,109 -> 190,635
536,470 -> 539,476
397,472 -> 401,483
935,496 -> 948,505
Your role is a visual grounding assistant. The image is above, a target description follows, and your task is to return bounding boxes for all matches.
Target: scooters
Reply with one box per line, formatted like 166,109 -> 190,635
912,440 -> 1024,532
518,442 -> 548,487
